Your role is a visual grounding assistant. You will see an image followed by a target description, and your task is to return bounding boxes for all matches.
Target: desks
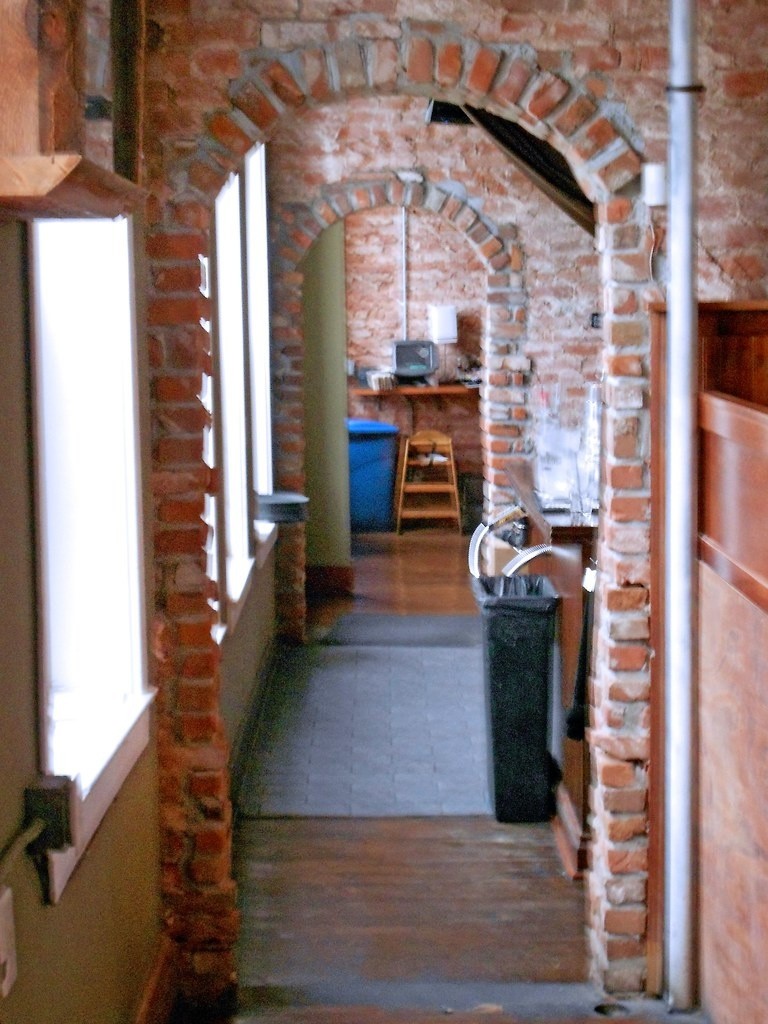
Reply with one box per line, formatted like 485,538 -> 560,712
357,383 -> 478,403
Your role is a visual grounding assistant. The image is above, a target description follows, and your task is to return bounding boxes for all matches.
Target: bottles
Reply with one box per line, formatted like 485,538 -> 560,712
455,349 -> 467,381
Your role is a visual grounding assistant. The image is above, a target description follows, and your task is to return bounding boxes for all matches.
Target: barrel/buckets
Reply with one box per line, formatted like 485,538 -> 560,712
346,416 -> 400,530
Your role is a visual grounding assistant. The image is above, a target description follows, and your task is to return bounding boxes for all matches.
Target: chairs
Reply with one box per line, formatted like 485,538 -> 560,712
395,430 -> 463,535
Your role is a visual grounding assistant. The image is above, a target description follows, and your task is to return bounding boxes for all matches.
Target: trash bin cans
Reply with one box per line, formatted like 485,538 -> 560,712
477,576 -> 561,824
347,418 -> 400,532
